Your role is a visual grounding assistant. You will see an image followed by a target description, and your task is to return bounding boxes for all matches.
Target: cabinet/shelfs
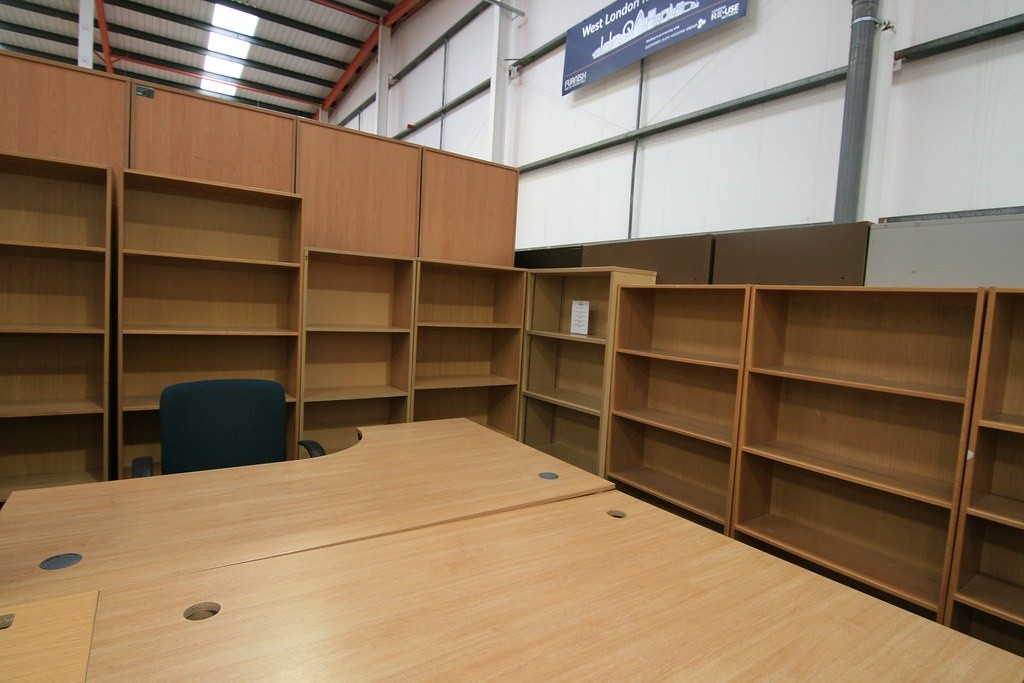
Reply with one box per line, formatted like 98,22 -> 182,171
0,49 -> 1024,658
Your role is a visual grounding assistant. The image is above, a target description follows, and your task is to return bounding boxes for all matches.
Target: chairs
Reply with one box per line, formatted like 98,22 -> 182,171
131,379 -> 327,478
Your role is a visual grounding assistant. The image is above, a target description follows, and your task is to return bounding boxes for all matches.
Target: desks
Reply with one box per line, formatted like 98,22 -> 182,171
86,487 -> 1024,683
0,590 -> 100,683
0,416 -> 616,611
